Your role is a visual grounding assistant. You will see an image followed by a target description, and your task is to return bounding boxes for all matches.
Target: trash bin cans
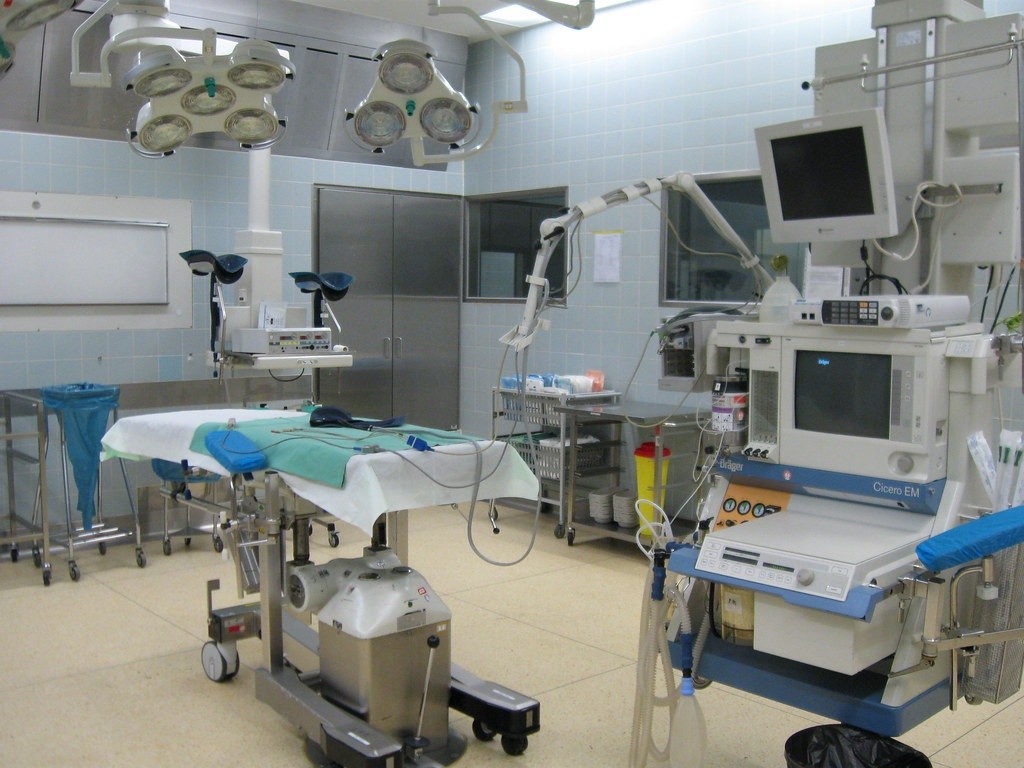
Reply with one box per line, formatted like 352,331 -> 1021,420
783,725 -> 933,768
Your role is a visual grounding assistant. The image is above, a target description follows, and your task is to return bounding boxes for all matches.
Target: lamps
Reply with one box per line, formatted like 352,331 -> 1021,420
25,0 -> 296,157
343,0 -> 595,166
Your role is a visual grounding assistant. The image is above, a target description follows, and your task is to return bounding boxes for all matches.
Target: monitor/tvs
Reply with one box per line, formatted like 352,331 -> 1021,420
781,336 -> 951,484
754,107 -> 898,244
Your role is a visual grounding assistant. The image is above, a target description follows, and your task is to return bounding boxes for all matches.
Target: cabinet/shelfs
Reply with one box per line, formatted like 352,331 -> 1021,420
488,386 -> 622,539
553,402 -> 712,558
0,390 -> 51,586
